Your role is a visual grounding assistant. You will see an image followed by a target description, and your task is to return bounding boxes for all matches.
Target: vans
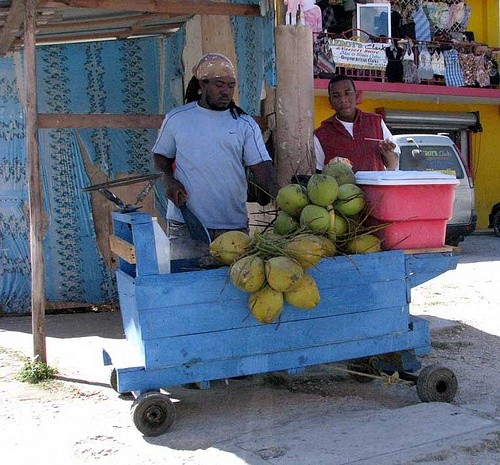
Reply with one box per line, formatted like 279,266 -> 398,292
388,133 -> 477,246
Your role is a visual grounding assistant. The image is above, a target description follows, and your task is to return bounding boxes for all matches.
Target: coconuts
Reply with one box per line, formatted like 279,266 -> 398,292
214,159 -> 380,324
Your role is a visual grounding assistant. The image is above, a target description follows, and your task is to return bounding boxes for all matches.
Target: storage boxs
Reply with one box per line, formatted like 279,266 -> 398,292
353,168 -> 462,250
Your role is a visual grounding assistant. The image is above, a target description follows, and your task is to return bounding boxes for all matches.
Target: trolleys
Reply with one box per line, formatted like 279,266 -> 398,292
81,173 -> 458,437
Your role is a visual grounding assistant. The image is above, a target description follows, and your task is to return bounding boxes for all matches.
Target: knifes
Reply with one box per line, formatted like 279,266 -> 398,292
178,194 -> 212,247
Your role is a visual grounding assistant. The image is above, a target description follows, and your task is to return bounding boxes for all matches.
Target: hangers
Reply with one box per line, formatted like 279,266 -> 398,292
388,34 -> 495,61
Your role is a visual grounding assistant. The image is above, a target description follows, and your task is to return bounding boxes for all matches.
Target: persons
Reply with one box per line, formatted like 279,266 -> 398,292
151,53 -> 280,261
312,75 -> 401,174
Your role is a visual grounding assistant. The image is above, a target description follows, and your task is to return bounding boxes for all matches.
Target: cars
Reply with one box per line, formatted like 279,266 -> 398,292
489,201 -> 500,237
